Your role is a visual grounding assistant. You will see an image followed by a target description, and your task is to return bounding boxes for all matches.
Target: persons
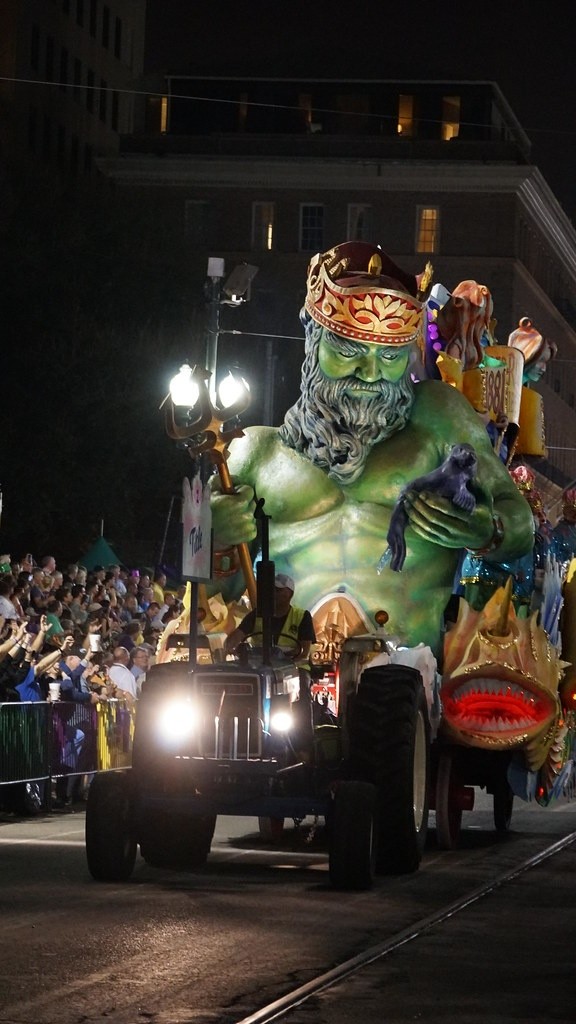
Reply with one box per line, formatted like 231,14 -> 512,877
508,326 -> 558,386
223,574 -> 317,661
0,554 -> 186,818
206,240 -> 536,677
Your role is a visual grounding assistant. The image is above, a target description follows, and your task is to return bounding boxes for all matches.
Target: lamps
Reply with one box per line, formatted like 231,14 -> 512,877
311,121 -> 323,133
168,356 -> 251,411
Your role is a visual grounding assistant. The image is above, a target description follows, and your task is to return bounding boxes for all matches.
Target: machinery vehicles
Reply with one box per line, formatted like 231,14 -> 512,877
85,505 -> 446,887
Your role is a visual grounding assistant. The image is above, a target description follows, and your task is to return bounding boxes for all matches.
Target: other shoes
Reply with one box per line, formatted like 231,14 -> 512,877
53,795 -> 73,809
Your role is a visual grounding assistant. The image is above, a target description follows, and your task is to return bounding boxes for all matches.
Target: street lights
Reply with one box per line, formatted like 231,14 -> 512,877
162,277 -> 253,658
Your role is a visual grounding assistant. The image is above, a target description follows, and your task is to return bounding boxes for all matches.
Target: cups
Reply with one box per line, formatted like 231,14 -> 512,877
49,682 -> 61,700
89,634 -> 101,652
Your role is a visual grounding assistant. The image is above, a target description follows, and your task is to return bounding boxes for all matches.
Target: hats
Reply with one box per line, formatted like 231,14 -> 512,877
68,644 -> 86,659
275,574 -> 295,590
26,624 -> 41,634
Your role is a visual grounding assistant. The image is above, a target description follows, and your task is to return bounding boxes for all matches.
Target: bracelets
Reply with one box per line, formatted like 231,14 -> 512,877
464,513 -> 505,557
58,648 -> 66,655
13,637 -> 19,645
23,642 -> 29,645
